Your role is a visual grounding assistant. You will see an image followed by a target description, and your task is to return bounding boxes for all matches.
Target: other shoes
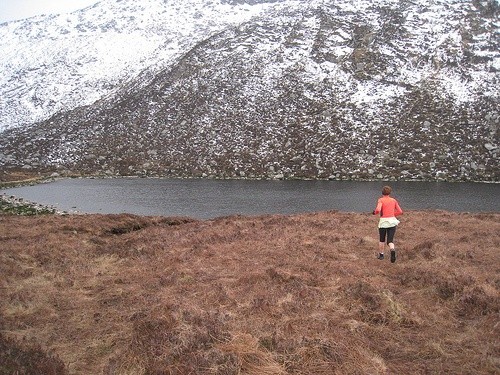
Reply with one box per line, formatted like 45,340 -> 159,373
390,249 -> 396,262
377,253 -> 384,260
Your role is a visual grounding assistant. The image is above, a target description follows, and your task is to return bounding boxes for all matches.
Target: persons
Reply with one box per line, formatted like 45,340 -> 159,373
372,186 -> 402,263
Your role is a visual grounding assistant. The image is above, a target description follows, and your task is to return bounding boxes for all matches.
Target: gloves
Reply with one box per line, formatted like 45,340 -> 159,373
372,210 -> 376,214
